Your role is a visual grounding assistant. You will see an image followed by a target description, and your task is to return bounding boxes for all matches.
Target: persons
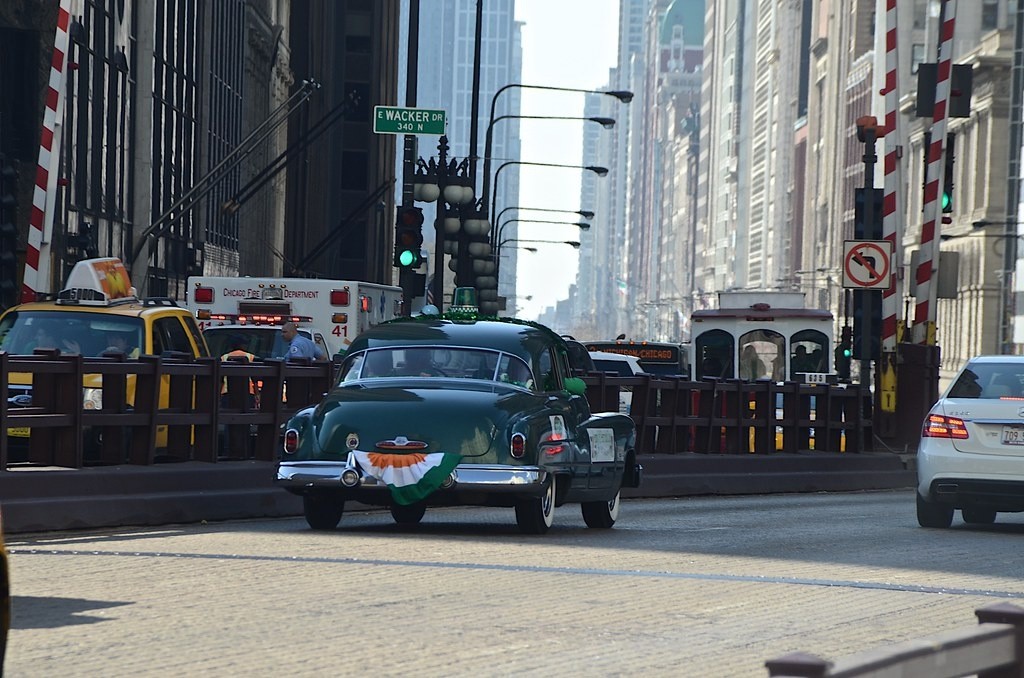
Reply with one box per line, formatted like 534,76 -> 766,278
281,322 -> 327,364
25,324 -> 62,355
548,416 -> 568,440
63,332 -> 138,358
790,346 -> 835,383
740,345 -> 766,381
218,337 -> 261,412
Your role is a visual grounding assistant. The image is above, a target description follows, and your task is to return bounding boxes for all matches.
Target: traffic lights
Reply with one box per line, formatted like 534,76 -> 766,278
841,327 -> 852,357
924,133 -> 953,213
394,206 -> 423,268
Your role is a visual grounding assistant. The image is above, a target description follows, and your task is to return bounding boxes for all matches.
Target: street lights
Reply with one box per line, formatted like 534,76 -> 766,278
415,83 -> 633,315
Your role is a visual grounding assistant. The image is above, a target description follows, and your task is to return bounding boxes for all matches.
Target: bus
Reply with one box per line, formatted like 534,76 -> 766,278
560,293 -> 846,454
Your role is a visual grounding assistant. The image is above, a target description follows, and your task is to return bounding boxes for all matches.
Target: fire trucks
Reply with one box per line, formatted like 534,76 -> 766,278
184,274 -> 404,352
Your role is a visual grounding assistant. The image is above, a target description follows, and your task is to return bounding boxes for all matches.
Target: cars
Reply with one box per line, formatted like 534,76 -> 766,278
274,287 -> 643,533
916,356 -> 1024,528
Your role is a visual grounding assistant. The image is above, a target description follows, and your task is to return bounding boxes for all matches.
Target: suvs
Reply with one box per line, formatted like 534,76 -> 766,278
1,258 -> 333,462
